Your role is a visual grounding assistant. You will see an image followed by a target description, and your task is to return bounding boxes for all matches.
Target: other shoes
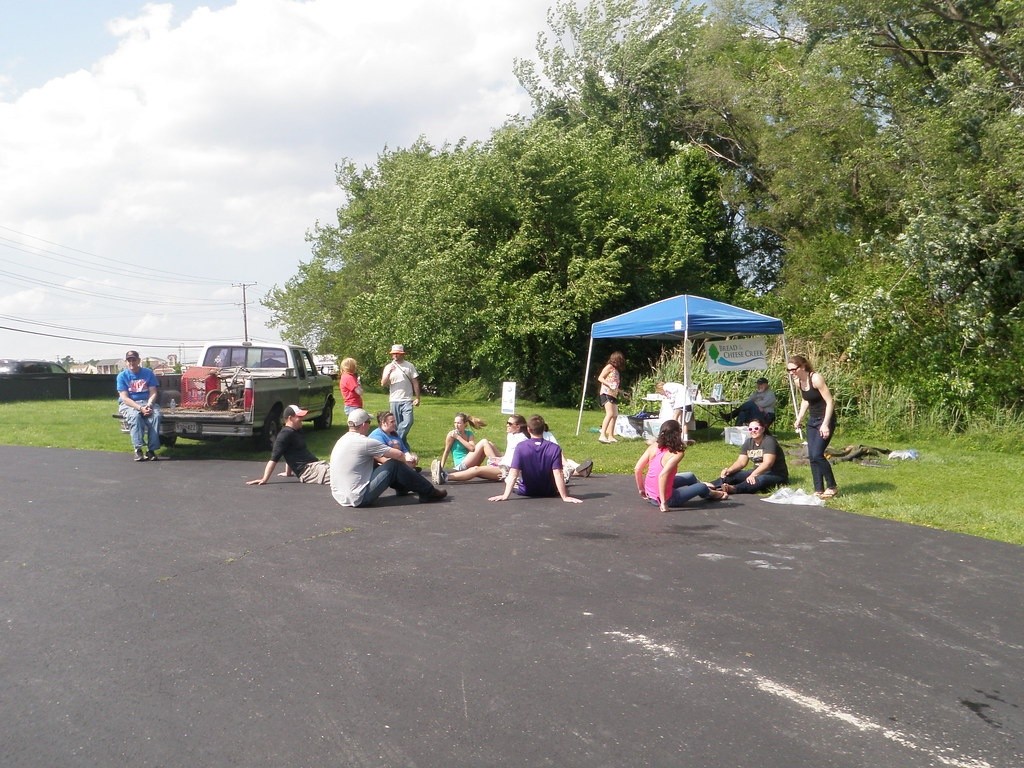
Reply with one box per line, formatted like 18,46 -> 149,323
144,450 -> 157,461
719,412 -> 731,423
598,436 -> 619,444
394,487 -> 408,496
413,466 -> 423,473
419,486 -> 447,502
430,459 -> 448,485
576,459 -> 593,477
133,449 -> 144,461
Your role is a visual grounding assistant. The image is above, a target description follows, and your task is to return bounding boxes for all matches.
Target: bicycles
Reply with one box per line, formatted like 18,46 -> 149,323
205,374 -> 244,410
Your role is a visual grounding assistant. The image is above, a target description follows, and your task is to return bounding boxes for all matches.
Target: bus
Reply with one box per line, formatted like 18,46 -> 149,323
0,359 -> 20,374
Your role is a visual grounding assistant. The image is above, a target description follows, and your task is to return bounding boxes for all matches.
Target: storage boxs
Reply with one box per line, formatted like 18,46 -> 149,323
643,419 -> 669,438
724,426 -> 751,445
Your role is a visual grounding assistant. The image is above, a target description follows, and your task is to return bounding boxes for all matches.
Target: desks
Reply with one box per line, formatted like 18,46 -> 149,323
641,398 -> 742,441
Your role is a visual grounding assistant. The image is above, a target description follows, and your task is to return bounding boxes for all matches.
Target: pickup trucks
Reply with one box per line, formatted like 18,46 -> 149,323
306,361 -> 339,378
121,340 -> 340,451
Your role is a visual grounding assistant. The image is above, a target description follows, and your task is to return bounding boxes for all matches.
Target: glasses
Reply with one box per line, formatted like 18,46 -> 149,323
747,426 -> 760,432
786,366 -> 799,373
506,421 -> 518,426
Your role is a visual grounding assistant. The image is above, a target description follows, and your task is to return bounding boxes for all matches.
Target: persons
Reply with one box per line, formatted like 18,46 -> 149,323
633,421 -> 728,511
116,351 -> 161,461
719,378 -> 775,428
330,409 -> 447,508
786,356 -> 838,499
339,358 -> 363,416
431,412 -> 584,504
382,345 -> 420,452
656,381 -> 692,442
597,351 -> 626,443
246,405 -> 330,485
704,419 -> 788,493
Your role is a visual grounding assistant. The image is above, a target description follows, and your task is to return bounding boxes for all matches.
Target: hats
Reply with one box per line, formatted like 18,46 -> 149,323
754,378 -> 768,384
347,408 -> 374,426
125,350 -> 139,360
283,405 -> 309,421
389,344 -> 406,354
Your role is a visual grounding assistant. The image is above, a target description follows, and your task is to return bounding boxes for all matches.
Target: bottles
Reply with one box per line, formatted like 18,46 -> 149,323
170,399 -> 176,414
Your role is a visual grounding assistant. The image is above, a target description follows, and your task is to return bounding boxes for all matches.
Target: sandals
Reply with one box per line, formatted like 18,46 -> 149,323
720,483 -> 731,493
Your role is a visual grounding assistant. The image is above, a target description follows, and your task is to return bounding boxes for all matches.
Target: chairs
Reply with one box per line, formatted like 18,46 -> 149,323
732,404 -> 776,436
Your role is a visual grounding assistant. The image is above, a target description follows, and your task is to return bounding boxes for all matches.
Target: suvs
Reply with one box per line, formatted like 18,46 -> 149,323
18,360 -> 68,374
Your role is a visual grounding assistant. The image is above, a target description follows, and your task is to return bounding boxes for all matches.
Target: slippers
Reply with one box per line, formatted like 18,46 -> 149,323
812,491 -> 824,496
819,490 -> 839,499
708,490 -> 729,500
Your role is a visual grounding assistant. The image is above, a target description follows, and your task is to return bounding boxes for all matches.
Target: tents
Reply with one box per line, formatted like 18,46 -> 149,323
574,295 -> 804,446
